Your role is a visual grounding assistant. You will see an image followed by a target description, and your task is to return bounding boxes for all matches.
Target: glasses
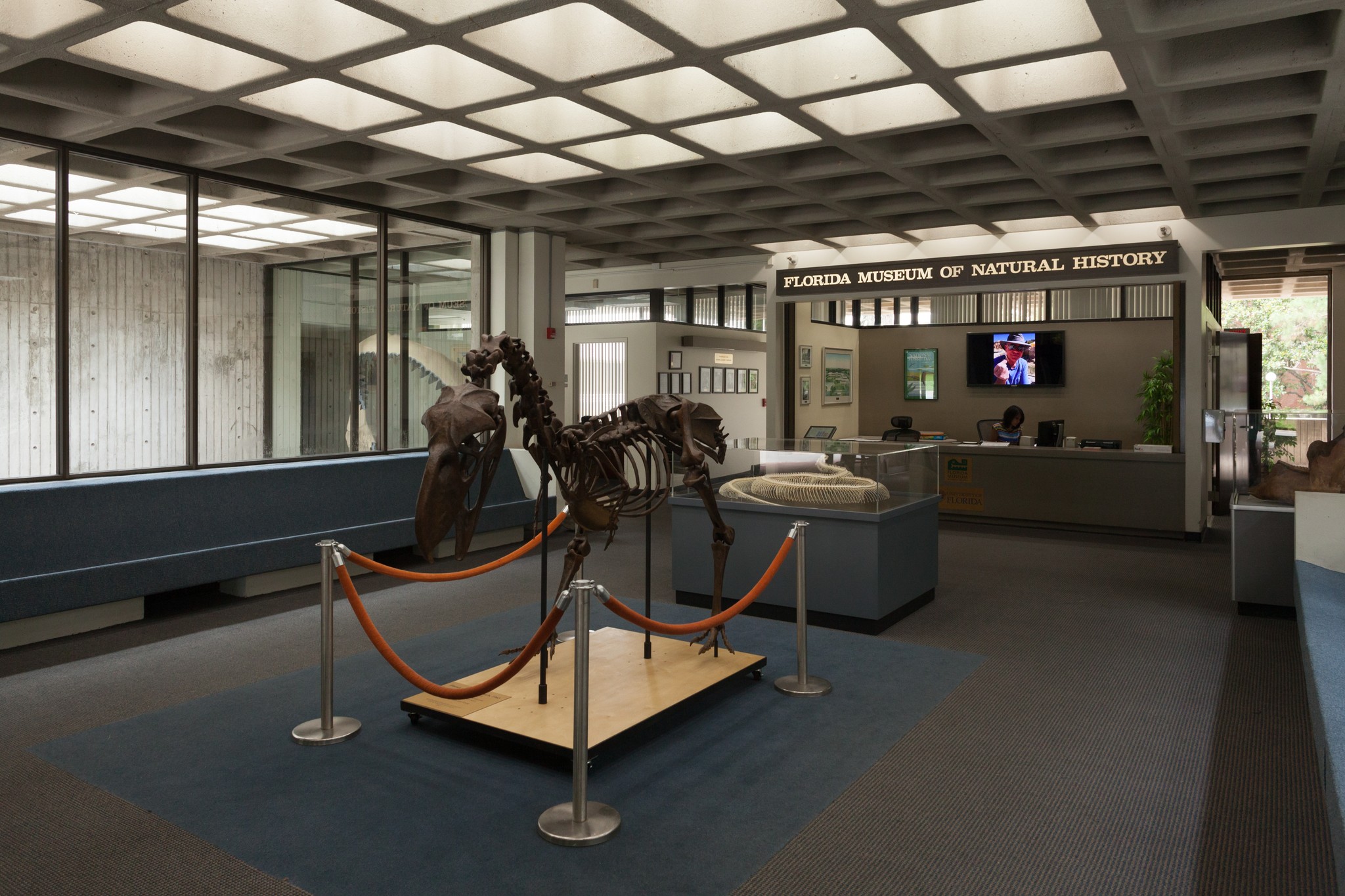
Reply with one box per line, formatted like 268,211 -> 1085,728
1006,344 -> 1025,351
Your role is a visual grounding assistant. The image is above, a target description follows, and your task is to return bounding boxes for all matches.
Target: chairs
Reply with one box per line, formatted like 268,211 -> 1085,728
881,416 -> 920,443
977,419 -> 1002,443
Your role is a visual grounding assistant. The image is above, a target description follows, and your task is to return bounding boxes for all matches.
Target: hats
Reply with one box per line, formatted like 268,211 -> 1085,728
1000,333 -> 1031,351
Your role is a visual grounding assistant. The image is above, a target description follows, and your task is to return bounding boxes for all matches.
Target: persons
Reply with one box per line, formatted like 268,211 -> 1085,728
989,405 -> 1025,445
993,333 -> 1032,384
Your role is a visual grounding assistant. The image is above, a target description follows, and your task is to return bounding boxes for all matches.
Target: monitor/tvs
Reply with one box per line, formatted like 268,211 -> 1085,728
966,329 -> 1065,388
1036,419 -> 1064,447
803,426 -> 837,439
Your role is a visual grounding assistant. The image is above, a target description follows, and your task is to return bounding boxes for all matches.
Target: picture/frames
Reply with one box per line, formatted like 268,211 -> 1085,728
658,372 -> 692,395
904,349 -> 939,400
799,345 -> 812,368
800,377 -> 811,405
699,366 -> 759,394
822,346 -> 853,405
669,351 -> 683,370
